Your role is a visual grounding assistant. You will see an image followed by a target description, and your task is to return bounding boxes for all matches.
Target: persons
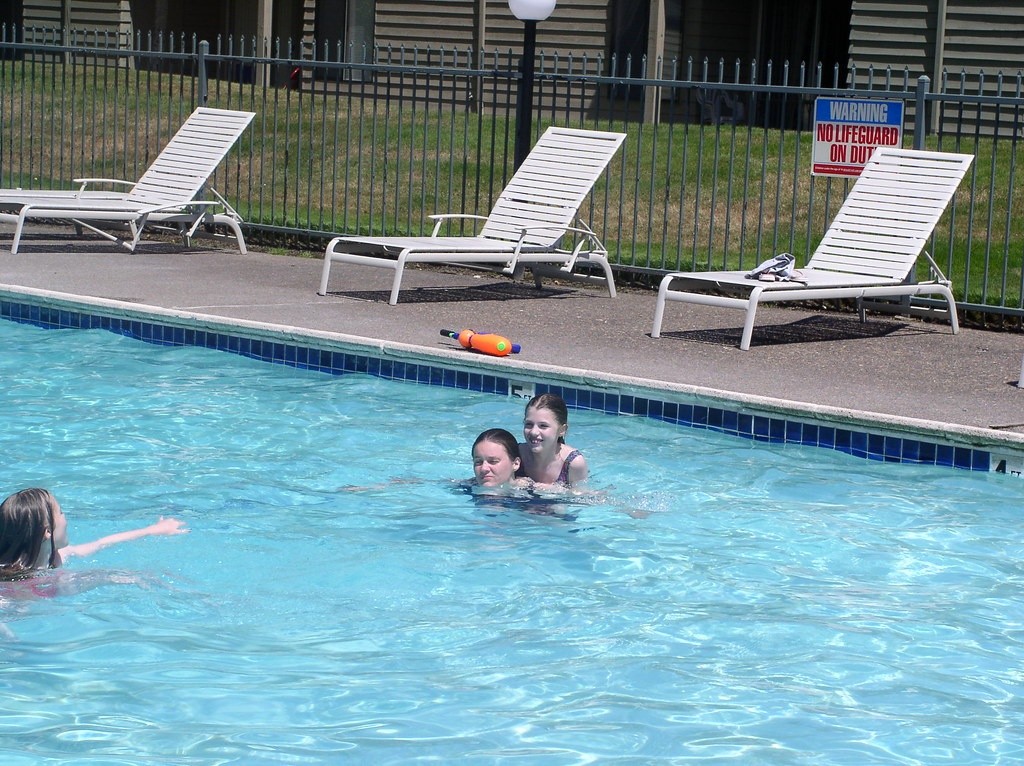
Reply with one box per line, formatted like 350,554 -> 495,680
468,395 -> 588,491
0,487 -> 71,579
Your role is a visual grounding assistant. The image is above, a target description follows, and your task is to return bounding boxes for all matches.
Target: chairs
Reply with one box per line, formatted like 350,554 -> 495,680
319,126 -> 628,304
651,147 -> 975,349
0,106 -> 256,254
